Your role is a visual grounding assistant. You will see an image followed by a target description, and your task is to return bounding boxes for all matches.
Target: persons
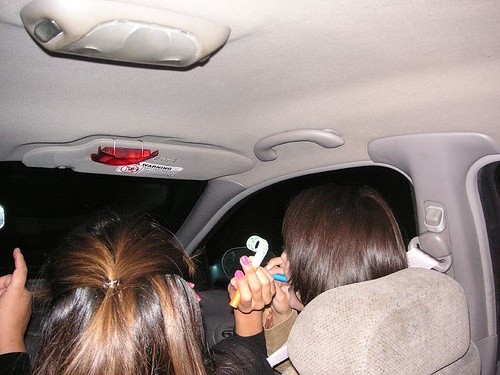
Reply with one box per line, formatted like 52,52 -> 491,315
0,247 -> 38,375
30,211 -> 272,375
259,187 -> 407,375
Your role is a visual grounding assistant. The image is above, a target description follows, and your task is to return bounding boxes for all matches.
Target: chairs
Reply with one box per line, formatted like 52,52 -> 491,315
280,265 -> 482,375
